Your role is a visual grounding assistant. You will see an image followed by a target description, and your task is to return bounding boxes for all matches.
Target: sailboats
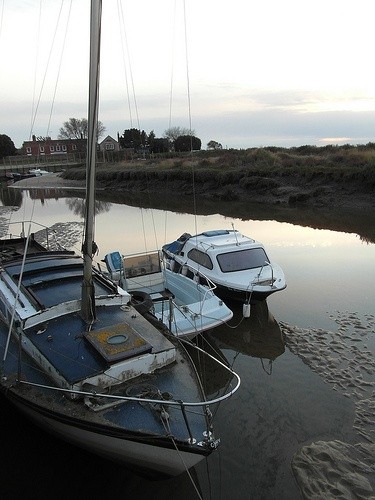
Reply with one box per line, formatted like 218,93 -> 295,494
0,1 -> 241,479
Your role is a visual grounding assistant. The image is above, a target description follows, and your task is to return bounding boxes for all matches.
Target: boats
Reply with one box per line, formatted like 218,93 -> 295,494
99,250 -> 234,342
161,222 -> 288,305
4,168 -> 48,182
